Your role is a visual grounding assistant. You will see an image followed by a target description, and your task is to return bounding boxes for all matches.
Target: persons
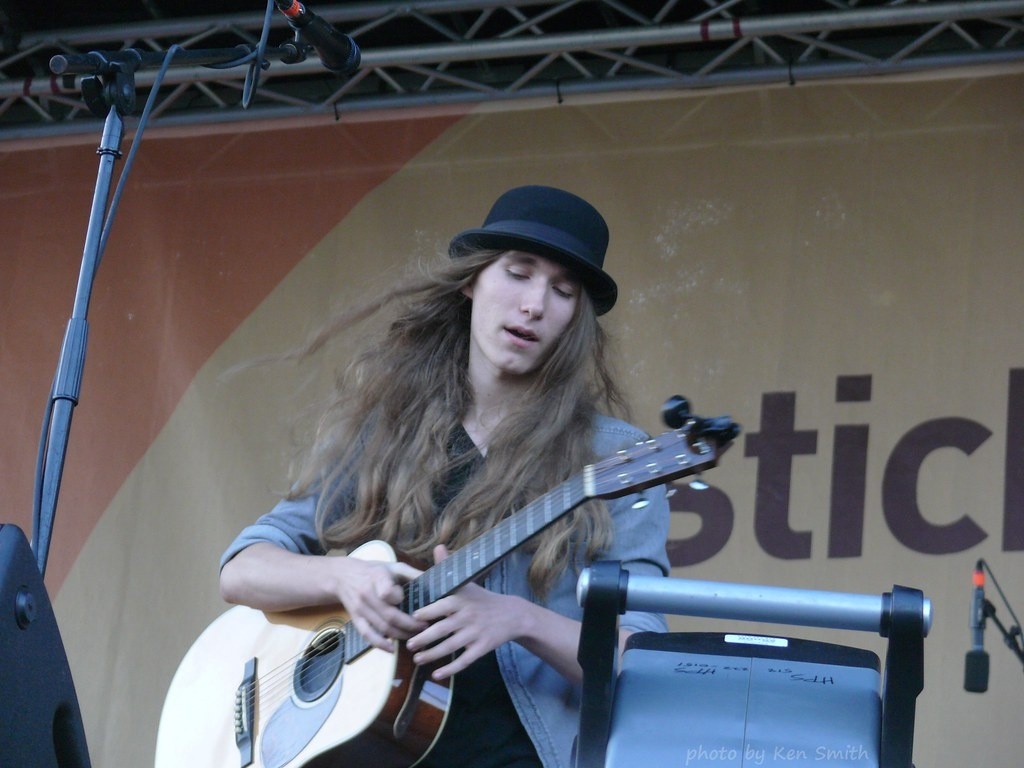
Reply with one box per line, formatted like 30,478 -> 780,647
214,180 -> 672,768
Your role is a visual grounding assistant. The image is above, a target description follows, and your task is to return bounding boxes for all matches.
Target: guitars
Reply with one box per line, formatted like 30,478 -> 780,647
154,395 -> 741,768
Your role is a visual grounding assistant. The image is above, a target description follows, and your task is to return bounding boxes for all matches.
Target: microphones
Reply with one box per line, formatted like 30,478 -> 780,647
274,0 -> 362,73
963,560 -> 989,692
49,48 -> 148,75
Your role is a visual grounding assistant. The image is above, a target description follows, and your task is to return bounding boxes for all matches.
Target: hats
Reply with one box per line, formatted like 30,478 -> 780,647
448,183 -> 618,317
